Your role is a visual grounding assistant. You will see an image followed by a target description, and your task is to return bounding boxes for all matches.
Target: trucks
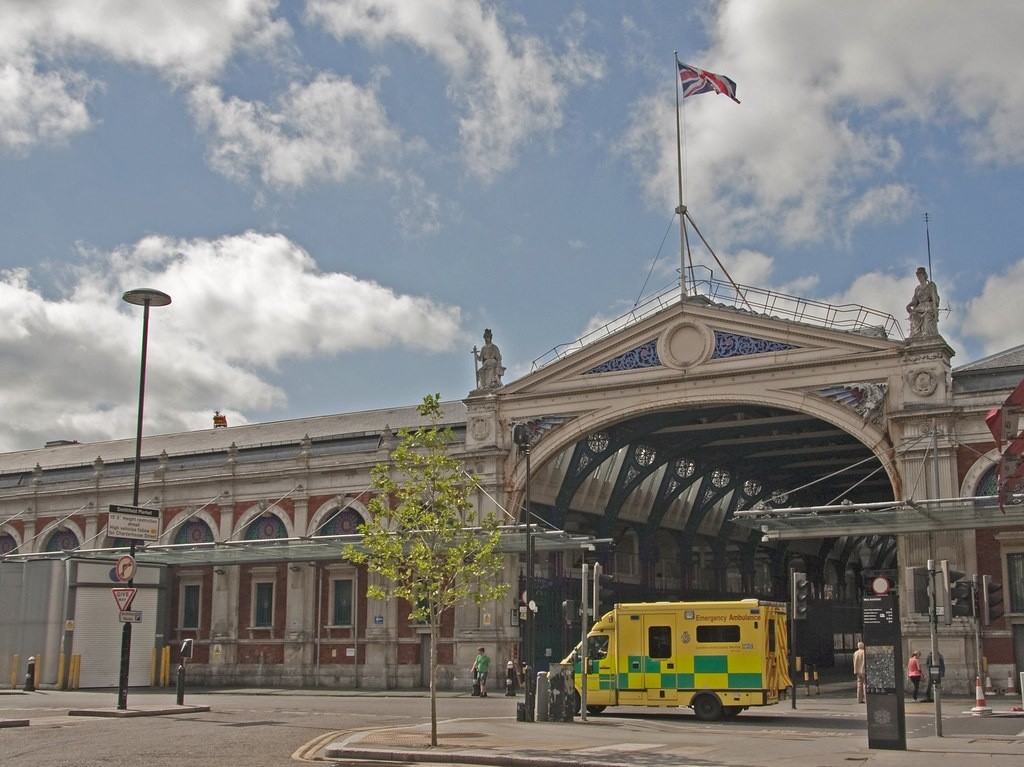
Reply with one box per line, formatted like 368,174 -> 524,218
548,599 -> 792,722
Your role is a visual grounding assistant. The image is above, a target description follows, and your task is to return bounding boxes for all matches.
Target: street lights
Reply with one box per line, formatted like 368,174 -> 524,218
117,286 -> 172,706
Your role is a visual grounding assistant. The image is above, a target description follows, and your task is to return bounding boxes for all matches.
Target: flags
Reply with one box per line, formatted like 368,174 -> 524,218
677,60 -> 741,103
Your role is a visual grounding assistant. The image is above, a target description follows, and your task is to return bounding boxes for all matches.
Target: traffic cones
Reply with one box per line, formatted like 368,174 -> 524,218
971,676 -> 992,715
984,672 -> 997,696
1005,671 -> 1019,696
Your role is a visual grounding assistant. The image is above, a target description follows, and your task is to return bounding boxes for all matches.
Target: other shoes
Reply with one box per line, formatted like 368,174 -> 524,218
479,693 -> 487,697
913,699 -> 918,703
859,701 -> 866,704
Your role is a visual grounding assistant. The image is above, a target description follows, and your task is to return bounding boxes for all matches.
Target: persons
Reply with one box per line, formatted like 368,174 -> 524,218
906,267 -> 941,339
470,646 -> 491,698
921,652 -> 945,702
471,328 -> 507,390
852,641 -> 866,703
908,651 -> 925,702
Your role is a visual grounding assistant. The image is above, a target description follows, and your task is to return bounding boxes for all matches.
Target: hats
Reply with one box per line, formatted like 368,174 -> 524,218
478,647 -> 484,651
